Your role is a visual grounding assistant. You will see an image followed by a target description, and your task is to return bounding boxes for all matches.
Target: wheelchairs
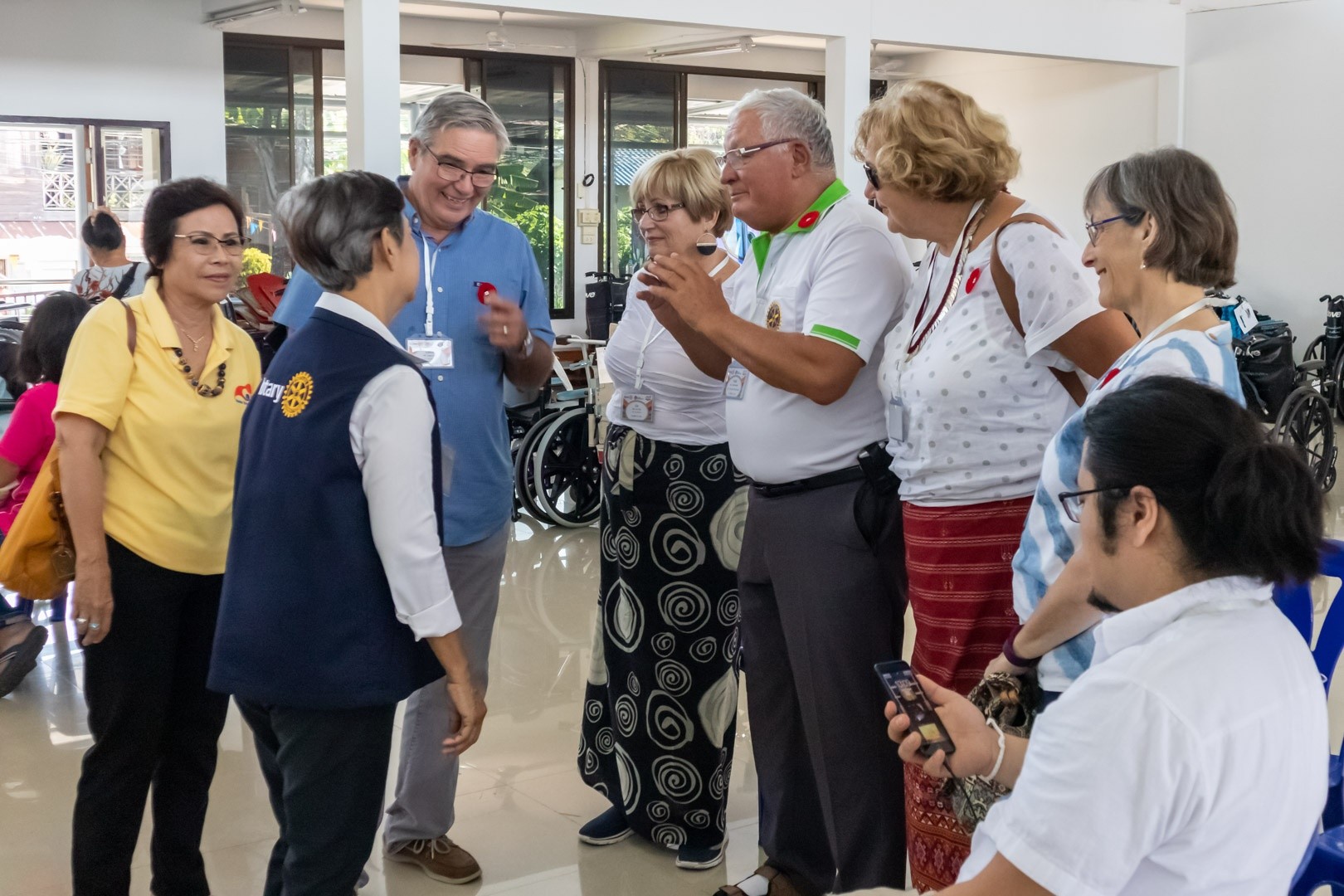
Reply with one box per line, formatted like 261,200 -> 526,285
503,336 -> 607,530
1224,294 -> 1344,494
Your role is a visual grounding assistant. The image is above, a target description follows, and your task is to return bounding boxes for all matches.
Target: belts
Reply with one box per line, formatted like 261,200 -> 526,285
745,464 -> 867,498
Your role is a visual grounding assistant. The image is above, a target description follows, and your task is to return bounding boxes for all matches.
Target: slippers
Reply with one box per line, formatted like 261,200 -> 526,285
0,625 -> 48,698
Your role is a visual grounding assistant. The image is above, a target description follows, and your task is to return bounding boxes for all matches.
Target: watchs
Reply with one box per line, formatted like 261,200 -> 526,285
505,329 -> 534,362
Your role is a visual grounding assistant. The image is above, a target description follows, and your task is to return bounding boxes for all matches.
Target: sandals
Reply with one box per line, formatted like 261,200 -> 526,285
713,863 -> 803,896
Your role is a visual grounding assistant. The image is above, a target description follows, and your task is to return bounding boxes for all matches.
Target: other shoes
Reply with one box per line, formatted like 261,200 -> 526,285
674,828 -> 730,870
382,833 -> 482,884
578,805 -> 637,846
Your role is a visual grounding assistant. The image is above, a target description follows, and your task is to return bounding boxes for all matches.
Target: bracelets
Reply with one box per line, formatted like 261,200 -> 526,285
1002,623 -> 1041,667
976,718 -> 1005,785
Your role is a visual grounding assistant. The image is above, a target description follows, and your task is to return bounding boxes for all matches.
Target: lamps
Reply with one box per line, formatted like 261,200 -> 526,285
203,0 -> 307,29
644,36 -> 755,61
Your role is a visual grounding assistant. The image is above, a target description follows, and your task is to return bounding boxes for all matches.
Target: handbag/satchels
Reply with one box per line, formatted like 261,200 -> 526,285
0,437 -> 78,600
938,673 -> 1041,837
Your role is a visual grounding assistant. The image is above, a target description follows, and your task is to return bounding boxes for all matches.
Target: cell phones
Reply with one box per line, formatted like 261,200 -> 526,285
875,660 -> 955,757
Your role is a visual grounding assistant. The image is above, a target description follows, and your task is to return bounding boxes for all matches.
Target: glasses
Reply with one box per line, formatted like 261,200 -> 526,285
425,145 -> 499,188
174,234 -> 252,256
1058,485 -> 1128,523
714,137 -> 812,172
862,163 -> 892,190
1084,214 -> 1137,248
630,203 -> 685,223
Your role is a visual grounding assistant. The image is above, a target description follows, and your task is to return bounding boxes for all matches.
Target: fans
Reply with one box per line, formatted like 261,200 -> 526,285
431,11 -> 576,52
811,40 -> 913,77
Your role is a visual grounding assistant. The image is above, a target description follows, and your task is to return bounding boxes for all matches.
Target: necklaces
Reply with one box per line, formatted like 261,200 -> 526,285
171,317 -> 209,351
173,347 -> 227,398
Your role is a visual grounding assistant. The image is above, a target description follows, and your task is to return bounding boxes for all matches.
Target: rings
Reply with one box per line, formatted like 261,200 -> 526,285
503,326 -> 507,335
75,618 -> 89,626
88,624 -> 100,631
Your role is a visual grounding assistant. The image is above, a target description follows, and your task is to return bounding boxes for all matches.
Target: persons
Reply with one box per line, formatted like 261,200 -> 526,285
272,90 -> 555,881
574,81 -> 1326,896
0,172 -> 489,896
